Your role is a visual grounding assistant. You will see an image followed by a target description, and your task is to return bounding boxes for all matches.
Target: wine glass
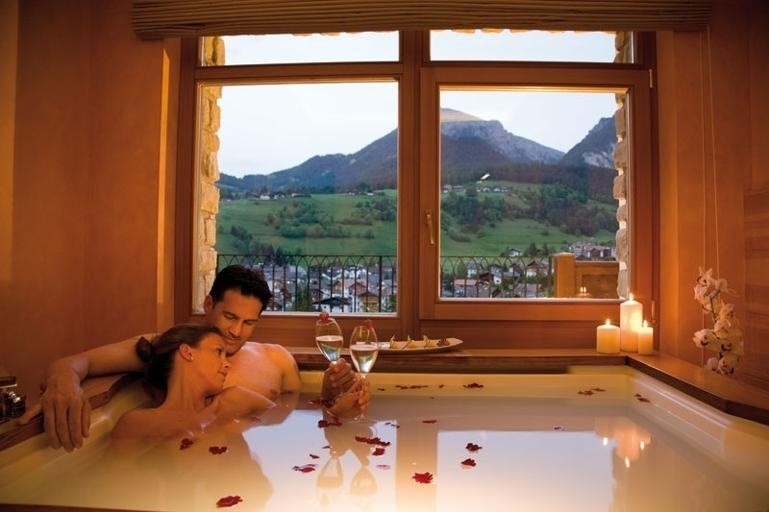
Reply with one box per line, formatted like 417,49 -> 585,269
349,326 -> 378,425
315,318 -> 344,402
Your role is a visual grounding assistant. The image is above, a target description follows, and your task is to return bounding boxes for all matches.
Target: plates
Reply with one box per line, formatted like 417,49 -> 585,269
356,337 -> 464,352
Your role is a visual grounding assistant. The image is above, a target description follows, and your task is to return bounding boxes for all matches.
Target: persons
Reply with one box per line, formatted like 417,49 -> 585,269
17,264 -> 358,453
111,323 -> 370,439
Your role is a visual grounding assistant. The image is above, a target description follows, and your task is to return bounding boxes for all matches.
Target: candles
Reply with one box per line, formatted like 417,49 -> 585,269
595,291 -> 654,356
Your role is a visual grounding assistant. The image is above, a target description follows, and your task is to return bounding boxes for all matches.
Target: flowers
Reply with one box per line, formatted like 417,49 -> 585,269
692,261 -> 747,377
